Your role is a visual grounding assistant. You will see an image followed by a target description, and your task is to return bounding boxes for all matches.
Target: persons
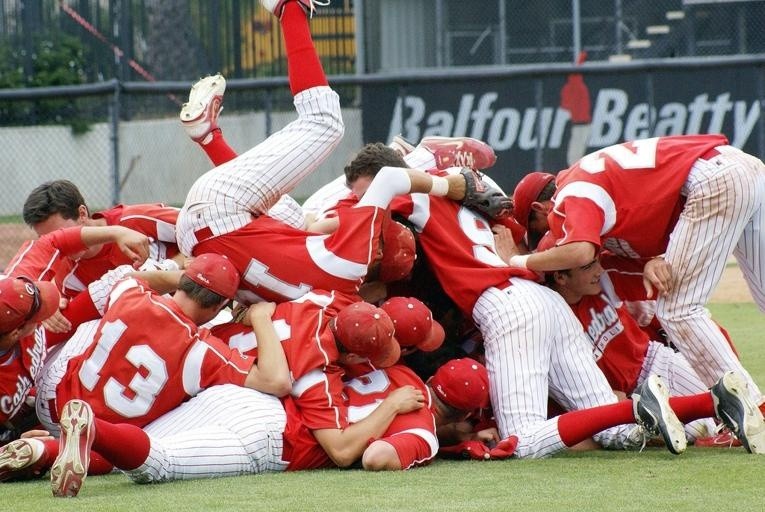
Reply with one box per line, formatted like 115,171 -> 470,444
1,2 -> 765,495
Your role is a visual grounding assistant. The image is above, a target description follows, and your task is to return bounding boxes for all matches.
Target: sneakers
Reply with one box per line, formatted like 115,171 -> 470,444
0,435 -> 49,482
709,371 -> 765,455
180,72 -> 226,144
259,0 -> 331,18
626,374 -> 688,455
388,135 -> 417,158
695,423 -> 742,447
416,136 -> 497,171
49,399 -> 96,496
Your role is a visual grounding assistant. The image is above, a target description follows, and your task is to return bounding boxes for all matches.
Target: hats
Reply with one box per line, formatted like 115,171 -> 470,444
379,204 -> 418,285
334,301 -> 401,369
0,276 -> 59,334
536,229 -> 604,274
512,172 -> 556,252
431,357 -> 490,410
381,296 -> 445,352
184,251 -> 240,300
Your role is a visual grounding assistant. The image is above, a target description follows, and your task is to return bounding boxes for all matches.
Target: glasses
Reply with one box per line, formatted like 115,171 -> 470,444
16,275 -> 40,321
